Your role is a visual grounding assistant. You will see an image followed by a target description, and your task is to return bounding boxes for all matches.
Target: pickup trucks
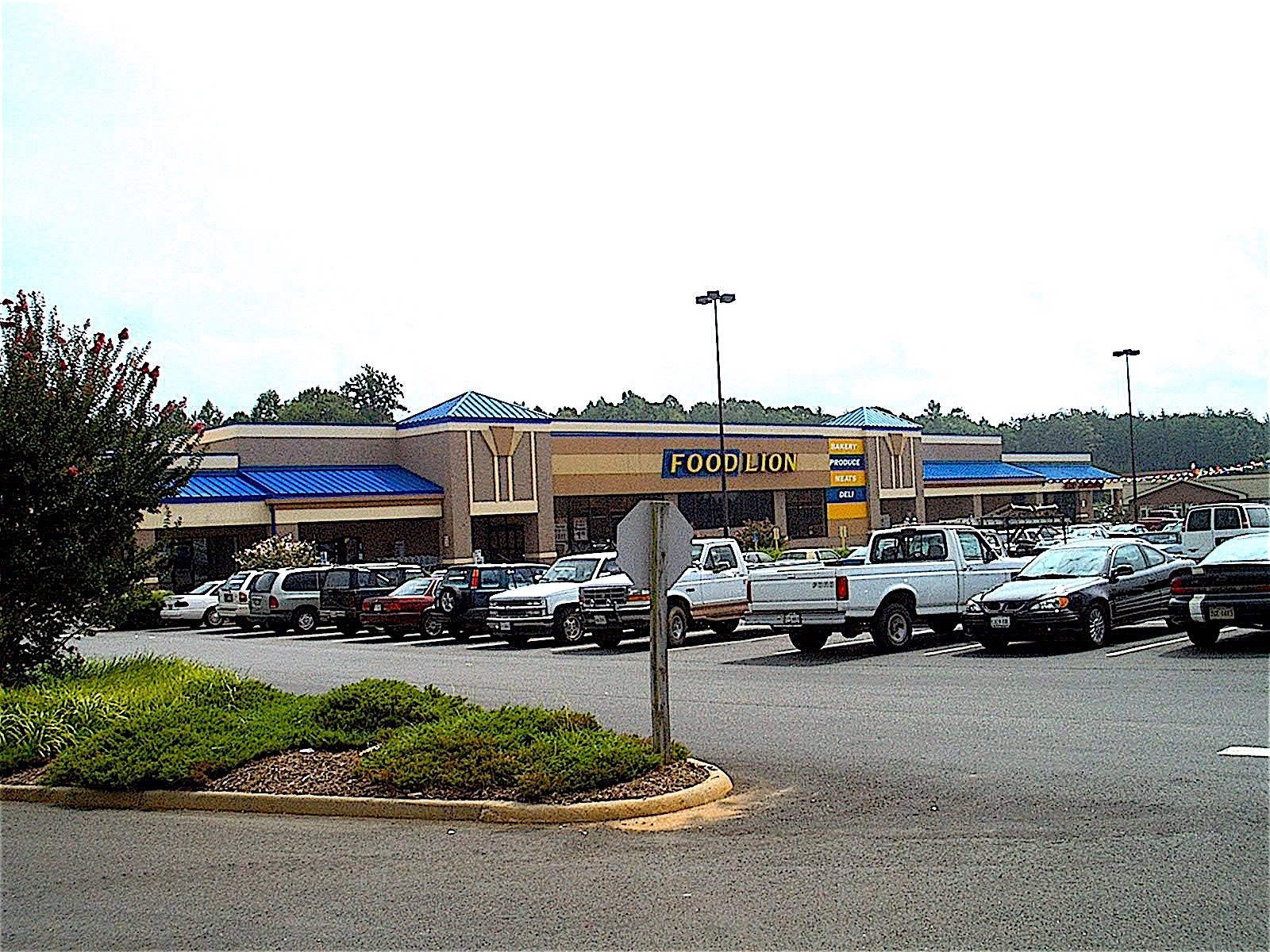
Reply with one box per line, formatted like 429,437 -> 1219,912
486,551 -> 623,648
575,537 -> 826,651
741,522 -> 1036,654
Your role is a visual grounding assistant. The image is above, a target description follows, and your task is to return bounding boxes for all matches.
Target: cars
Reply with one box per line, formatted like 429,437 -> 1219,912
159,579 -> 244,629
1166,530 -> 1270,649
358,575 -> 464,640
960,536 -> 1197,651
739,499 -> 1270,569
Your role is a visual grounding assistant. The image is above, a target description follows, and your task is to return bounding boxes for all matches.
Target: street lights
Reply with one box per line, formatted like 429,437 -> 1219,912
696,290 -> 737,537
1113,349 -> 1141,522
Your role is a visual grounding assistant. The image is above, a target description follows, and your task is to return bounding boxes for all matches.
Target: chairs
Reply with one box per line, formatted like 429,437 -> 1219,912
928,545 -> 943,559
880,546 -> 899,560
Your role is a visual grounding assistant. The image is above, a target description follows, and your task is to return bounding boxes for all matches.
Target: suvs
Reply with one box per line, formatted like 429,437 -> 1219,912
317,562 -> 433,635
215,565 -> 278,631
246,566 -> 335,635
430,562 -> 552,641
